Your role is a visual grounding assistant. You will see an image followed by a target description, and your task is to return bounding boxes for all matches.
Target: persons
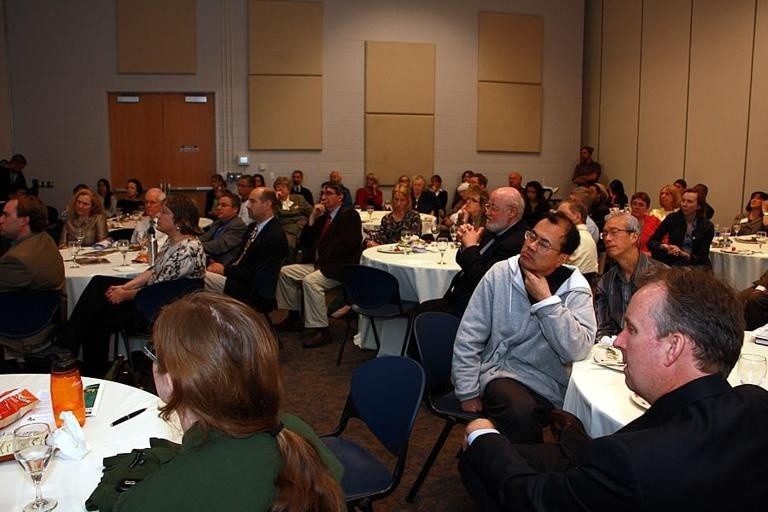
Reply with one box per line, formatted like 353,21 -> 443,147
87,293 -> 343,512
452,212 -> 598,457
355,146 -> 767,368
0,147 -> 364,374
456,269 -> 766,511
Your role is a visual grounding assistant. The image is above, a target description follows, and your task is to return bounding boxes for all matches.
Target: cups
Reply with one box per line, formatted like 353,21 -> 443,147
737,352 -> 767,389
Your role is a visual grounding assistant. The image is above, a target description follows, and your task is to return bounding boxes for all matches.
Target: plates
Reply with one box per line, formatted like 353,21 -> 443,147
591,345 -> 623,377
627,393 -> 650,414
377,245 -> 411,253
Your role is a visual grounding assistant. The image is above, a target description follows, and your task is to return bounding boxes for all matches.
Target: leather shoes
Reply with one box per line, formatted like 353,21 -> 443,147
274,317 -> 305,332
302,330 -> 333,348
547,407 -> 595,467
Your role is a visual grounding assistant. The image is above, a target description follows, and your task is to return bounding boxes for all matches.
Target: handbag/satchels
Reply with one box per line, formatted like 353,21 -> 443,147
104,353 -> 146,390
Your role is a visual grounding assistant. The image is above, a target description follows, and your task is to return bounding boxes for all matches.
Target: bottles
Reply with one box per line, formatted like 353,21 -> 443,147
145,227 -> 159,265
48,350 -> 87,431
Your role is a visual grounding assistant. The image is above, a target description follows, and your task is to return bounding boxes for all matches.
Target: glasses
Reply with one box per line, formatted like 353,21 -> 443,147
140,339 -> 162,371
319,191 -> 338,198
524,230 -> 564,254
236,181 -> 252,188
484,204 -> 512,215
599,227 -> 635,240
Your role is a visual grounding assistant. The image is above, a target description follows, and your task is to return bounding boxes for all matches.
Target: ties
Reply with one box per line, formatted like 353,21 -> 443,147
149,219 -> 155,233
233,227 -> 258,266
319,216 -> 332,240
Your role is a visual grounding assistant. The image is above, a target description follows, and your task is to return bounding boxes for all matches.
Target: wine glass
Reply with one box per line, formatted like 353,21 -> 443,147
395,225 -> 460,265
713,222 -> 767,255
354,204 -> 375,222
10,423 -> 57,512
68,226 -> 132,268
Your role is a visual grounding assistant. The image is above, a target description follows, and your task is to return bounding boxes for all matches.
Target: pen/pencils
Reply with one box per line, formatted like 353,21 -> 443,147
111,408 -> 148,426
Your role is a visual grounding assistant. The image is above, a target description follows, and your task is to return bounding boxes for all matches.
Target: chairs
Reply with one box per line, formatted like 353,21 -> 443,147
300,239 -> 361,340
336,264 -> 420,365
0,291 -> 60,373
115,279 -> 204,372
320,356 -> 426,512
248,262 -> 283,350
649,245 -> 689,267
404,312 -> 489,504
